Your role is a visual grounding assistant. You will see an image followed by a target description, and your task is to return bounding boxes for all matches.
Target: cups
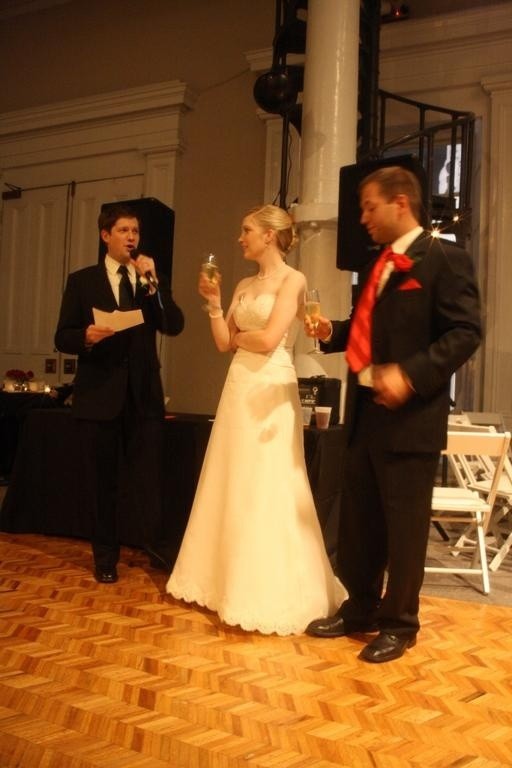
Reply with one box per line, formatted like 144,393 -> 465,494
314,406 -> 332,430
301,407 -> 313,425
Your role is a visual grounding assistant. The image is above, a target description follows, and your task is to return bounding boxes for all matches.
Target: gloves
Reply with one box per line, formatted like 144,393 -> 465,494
199,272 -> 223,318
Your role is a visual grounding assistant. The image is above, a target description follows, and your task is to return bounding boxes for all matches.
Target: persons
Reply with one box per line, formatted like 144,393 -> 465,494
305,166 -> 483,664
165,204 -> 349,636
55,203 -> 186,582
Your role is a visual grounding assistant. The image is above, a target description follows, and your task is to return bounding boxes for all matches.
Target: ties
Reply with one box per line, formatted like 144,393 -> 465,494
345,246 -> 415,374
118,266 -> 134,310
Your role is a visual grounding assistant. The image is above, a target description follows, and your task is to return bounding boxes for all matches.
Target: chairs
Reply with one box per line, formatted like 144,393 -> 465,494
425,409 -> 512,594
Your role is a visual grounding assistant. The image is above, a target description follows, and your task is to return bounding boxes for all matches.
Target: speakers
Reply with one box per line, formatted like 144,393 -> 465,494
335,152 -> 430,271
97,195 -> 175,316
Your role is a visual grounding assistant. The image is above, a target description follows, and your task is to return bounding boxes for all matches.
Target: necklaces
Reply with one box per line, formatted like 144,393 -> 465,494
257,261 -> 286,280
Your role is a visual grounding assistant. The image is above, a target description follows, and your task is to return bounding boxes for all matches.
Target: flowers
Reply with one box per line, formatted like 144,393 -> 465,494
5,369 -> 34,385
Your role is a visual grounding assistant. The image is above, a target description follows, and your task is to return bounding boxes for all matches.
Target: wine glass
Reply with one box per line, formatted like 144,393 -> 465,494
304,288 -> 325,356
199,252 -> 220,312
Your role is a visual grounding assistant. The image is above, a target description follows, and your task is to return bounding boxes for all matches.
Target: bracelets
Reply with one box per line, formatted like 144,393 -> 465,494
208,309 -> 224,319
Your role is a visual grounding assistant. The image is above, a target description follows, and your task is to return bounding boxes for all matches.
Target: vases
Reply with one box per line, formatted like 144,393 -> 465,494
14,386 -> 24,392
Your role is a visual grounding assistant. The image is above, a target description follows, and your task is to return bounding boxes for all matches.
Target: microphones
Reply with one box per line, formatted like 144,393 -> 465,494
129,248 -> 159,292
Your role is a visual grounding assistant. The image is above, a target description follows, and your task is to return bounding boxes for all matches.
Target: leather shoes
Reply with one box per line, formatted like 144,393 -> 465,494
307,616 -> 346,637
95,566 -> 117,583
360,633 -> 416,663
